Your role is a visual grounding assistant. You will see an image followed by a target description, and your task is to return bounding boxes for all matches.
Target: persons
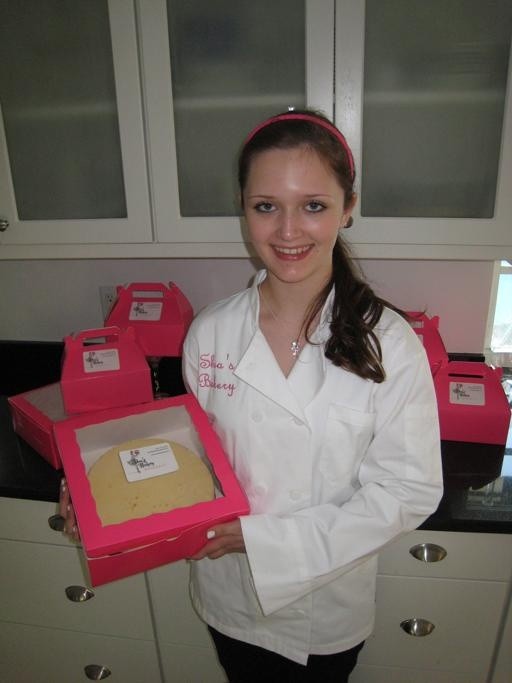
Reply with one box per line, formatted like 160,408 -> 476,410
179,109 -> 446,682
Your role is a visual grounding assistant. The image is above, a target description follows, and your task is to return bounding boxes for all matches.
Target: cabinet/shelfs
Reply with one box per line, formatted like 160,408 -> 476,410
148,531 -> 512,683
0,1 -> 154,266
1,494 -> 164,683
136,0 -> 511,261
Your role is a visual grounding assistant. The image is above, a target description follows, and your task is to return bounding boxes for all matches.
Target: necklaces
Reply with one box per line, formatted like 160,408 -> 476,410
259,283 -> 314,356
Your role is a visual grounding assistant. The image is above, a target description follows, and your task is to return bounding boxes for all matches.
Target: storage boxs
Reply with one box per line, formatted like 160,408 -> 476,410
433,360 -> 512,447
405,312 -> 450,373
104,280 -> 195,360
59,325 -> 153,416
8,380 -> 65,469
54,394 -> 250,589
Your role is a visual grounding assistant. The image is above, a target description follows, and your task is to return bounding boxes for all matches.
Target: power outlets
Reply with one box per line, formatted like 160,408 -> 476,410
102,286 -> 116,317
